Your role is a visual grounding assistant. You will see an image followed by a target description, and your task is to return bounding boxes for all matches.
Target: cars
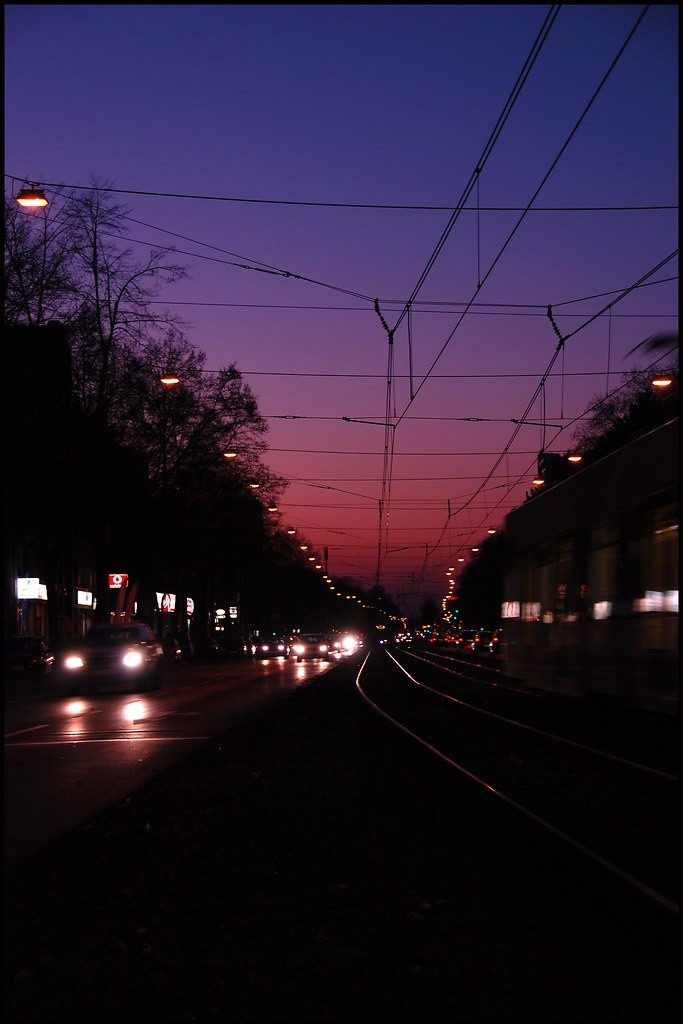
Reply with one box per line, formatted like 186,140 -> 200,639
4,635 -> 56,680
238,620 -> 510,662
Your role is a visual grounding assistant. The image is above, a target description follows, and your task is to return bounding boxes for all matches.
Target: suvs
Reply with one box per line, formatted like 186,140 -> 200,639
62,623 -> 166,694
